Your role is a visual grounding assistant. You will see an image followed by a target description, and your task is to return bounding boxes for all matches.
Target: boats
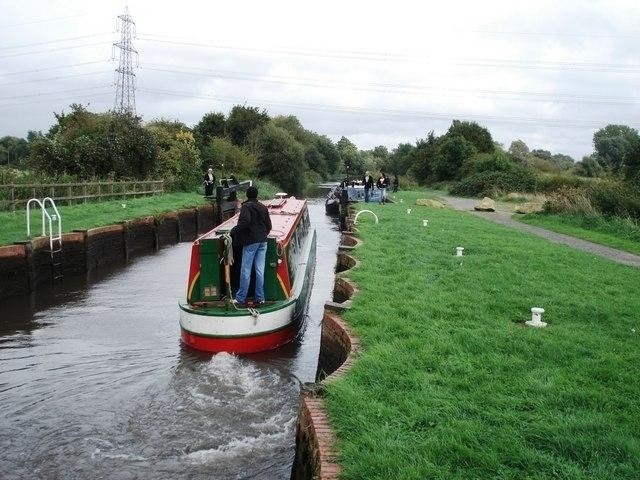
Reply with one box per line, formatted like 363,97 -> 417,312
325,183 -> 380,215
178,192 -> 316,355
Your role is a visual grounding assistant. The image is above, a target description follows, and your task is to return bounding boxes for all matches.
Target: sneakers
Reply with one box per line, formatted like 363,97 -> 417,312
229,299 -> 248,306
251,300 -> 265,308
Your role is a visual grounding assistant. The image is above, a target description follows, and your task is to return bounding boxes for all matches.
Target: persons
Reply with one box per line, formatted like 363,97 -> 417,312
377,174 -> 389,205
357,170 -> 375,203
231,185 -> 272,306
203,167 -> 216,195
385,175 -> 390,186
392,174 -> 399,193
